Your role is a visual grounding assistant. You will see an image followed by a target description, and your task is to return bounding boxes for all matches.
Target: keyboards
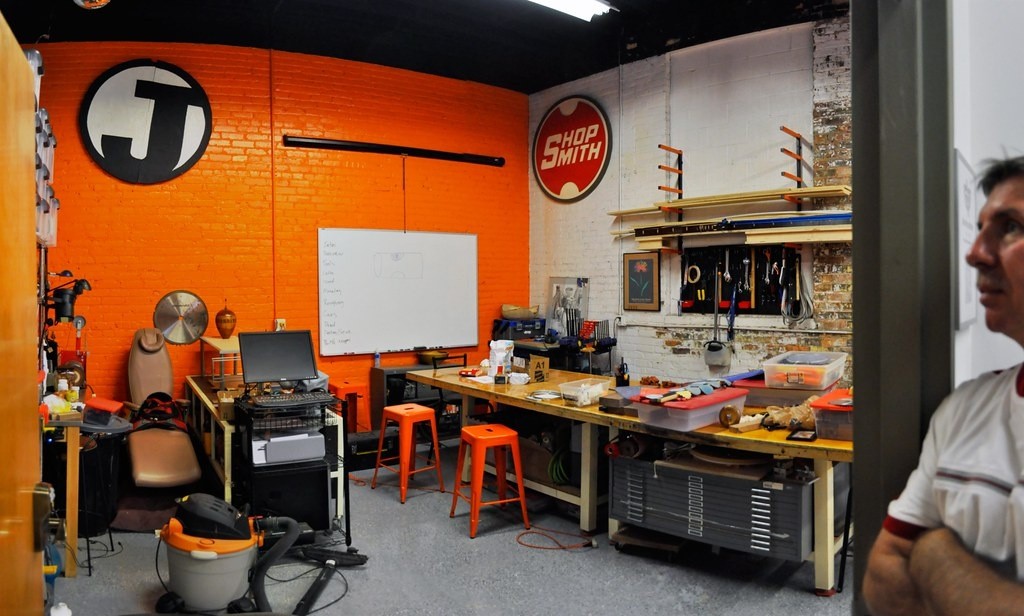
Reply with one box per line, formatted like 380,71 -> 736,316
254,391 -> 337,407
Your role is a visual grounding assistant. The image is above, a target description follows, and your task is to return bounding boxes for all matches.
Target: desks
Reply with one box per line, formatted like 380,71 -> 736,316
405,360 -> 856,598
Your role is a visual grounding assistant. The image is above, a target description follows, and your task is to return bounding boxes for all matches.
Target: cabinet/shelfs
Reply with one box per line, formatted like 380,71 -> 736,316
371,359 -> 471,445
185,372 -> 353,554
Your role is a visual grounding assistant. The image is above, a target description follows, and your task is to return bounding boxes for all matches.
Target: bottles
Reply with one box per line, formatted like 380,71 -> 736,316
374,350 -> 380,367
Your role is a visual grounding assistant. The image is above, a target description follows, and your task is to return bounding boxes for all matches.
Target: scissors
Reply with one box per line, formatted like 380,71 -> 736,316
616,357 -> 628,376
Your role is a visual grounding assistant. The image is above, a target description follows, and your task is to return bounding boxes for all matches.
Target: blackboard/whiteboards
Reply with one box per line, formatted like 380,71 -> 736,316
317,226 -> 479,357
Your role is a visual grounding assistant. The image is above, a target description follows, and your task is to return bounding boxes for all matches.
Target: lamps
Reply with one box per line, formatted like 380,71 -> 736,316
529,0 -> 620,23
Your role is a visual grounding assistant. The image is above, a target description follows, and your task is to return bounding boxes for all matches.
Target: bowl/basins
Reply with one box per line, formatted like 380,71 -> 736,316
500,302 -> 539,320
419,352 -> 448,365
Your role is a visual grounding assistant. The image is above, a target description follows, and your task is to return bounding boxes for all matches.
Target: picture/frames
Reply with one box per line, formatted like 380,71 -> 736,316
622,251 -> 661,312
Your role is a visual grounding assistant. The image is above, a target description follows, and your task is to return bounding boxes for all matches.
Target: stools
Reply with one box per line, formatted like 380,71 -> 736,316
369,402 -> 445,504
450,422 -> 531,539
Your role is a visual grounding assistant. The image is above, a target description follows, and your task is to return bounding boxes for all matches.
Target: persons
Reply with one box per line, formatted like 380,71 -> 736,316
860,152 -> 1024,616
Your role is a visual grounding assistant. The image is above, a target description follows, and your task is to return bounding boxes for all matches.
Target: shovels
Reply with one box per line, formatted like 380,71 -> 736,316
792,257 -> 802,316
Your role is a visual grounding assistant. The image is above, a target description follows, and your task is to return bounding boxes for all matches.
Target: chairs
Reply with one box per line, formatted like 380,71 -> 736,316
108,328 -> 209,533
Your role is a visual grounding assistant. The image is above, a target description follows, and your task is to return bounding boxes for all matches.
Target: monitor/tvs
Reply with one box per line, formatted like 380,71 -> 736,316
238,329 -> 319,384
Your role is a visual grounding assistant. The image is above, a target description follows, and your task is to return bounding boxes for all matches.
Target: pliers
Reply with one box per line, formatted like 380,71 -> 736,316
697,288 -> 706,314
